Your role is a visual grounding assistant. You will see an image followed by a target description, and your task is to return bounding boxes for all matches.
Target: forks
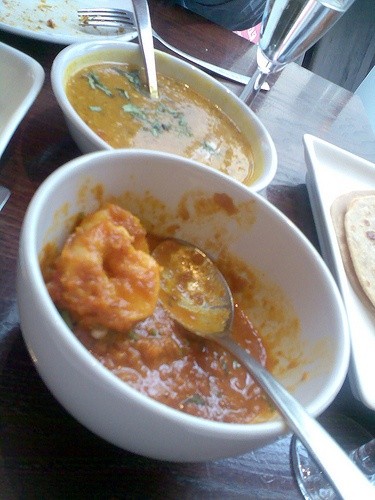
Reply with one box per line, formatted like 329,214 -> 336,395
77,7 -> 271,92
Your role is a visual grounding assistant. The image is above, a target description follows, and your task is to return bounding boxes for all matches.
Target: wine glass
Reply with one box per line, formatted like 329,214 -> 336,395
239,0 -> 356,108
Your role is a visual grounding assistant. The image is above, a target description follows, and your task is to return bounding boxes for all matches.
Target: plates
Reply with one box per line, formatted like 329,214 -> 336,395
0,0 -> 138,47
0,43 -> 46,159
302,133 -> 375,410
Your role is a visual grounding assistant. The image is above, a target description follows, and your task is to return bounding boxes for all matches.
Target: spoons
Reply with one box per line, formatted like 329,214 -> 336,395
150,237 -> 375,500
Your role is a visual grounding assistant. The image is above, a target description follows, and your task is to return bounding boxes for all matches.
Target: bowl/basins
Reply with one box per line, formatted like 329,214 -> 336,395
49,38 -> 278,193
14,148 -> 351,464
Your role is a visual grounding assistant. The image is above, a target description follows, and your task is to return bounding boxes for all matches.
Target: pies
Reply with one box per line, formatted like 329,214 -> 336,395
330,189 -> 375,314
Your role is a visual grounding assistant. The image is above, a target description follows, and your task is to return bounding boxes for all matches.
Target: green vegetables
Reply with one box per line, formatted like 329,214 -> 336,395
82,67 -> 215,155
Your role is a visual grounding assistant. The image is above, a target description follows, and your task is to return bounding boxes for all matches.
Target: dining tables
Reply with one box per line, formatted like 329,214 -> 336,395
0,1 -> 375,500
